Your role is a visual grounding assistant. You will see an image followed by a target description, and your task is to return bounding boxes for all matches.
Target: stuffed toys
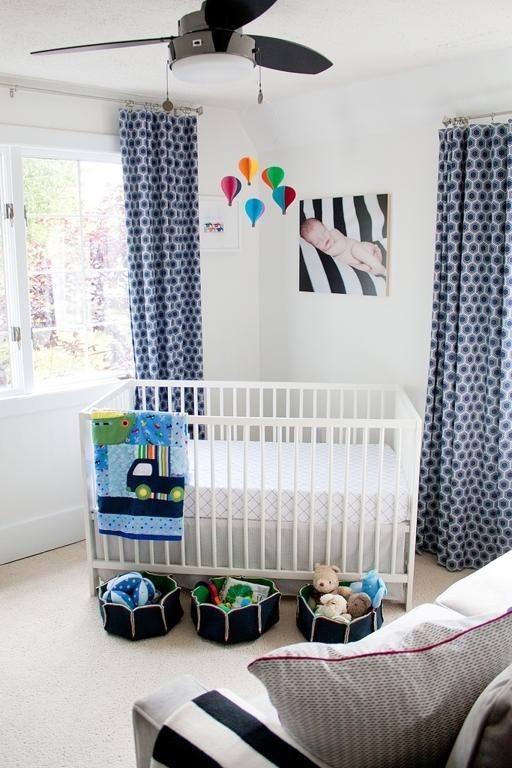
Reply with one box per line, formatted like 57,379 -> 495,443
308,562 -> 353,610
315,593 -> 353,624
330,586 -> 353,600
350,568 -> 387,608
346,592 -> 372,621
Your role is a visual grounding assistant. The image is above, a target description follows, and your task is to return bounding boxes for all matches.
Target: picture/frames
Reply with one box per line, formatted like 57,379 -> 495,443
198,193 -> 242,252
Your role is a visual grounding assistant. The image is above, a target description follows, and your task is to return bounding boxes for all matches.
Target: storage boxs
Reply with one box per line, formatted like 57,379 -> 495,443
96,569 -> 184,641
190,576 -> 282,646
295,580 -> 384,643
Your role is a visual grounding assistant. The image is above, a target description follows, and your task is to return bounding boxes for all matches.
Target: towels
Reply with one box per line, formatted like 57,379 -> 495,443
91,411 -> 187,541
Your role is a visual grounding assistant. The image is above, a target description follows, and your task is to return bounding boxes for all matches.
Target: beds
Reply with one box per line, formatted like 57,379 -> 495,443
78,378 -> 423,613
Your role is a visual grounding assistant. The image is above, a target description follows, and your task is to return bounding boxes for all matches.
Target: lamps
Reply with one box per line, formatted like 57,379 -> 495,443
166,0 -> 260,93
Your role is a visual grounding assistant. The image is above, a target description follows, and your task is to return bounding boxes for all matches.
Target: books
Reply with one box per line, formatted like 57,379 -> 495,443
219,577 -> 271,608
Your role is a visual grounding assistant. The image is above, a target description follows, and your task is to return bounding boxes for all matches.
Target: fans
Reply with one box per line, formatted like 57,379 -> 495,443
27,0 -> 333,113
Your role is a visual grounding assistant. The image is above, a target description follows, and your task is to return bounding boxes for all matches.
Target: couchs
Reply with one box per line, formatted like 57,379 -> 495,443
130,661 -> 512,767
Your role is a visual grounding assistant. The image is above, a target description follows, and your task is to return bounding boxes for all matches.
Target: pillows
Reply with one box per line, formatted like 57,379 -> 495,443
248,552 -> 512,768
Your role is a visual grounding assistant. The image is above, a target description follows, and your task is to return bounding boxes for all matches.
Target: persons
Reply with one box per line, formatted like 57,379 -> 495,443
300,218 -> 386,276
